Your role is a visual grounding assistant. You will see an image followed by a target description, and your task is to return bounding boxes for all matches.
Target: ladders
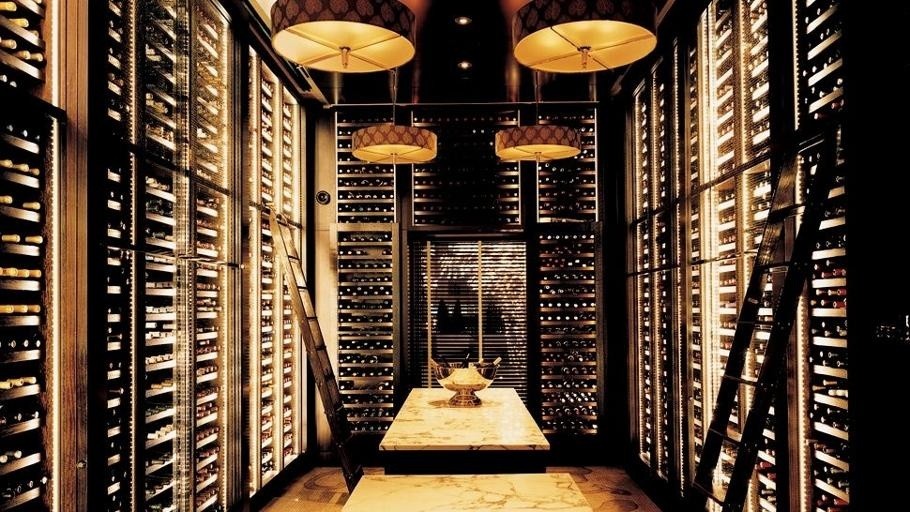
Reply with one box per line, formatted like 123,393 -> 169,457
268,209 -> 364,494
687,110 -> 839,512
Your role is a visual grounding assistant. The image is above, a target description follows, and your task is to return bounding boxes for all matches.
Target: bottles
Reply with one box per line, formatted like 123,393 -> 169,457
616,1 -> 910,512
332,107 -> 599,447
0,1 -> 326,512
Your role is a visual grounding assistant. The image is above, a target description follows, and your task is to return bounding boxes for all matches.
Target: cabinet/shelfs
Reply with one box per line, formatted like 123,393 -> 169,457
324,104 -> 601,448
607,0 -> 910,509
0,3 -> 314,511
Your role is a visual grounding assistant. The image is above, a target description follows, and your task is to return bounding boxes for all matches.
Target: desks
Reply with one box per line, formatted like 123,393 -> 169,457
337,387 -> 594,512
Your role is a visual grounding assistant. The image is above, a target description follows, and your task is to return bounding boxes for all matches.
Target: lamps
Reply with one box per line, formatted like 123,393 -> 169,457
269,0 -> 424,77
508,1 -> 660,81
